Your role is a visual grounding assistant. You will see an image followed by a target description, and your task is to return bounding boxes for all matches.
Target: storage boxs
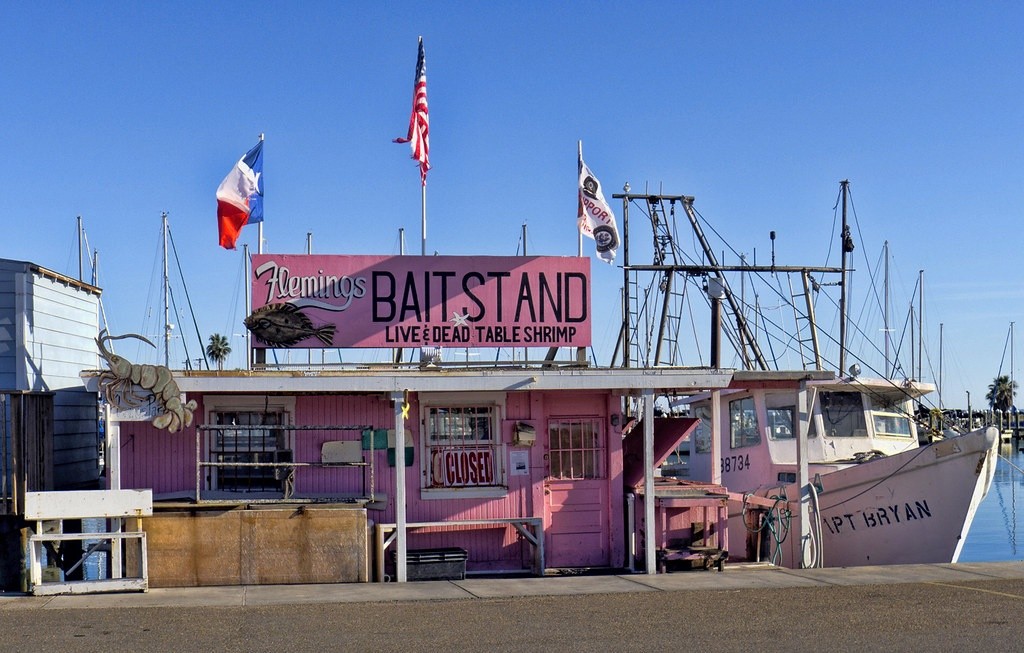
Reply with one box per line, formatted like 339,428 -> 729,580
388,545 -> 468,582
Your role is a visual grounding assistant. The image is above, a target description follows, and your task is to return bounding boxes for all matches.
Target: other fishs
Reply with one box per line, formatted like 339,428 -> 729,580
243,300 -> 339,351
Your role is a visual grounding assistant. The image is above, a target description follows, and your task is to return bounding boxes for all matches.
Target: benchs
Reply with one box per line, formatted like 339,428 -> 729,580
24,489 -> 153,595
375,515 -> 545,582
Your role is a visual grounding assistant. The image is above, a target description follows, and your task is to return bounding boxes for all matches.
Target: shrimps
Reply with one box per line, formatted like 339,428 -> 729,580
89,324 -> 200,436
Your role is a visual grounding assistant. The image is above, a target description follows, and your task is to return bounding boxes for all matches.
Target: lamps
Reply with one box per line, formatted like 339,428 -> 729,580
849,364 -> 861,380
421,345 -> 441,369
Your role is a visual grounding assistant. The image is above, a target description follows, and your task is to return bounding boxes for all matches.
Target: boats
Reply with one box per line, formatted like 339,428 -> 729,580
630,175 -> 1024,565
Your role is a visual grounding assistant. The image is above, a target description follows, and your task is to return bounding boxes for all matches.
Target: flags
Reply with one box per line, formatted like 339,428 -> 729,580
391,38 -> 432,186
216,140 -> 264,250
576,153 -> 621,264
